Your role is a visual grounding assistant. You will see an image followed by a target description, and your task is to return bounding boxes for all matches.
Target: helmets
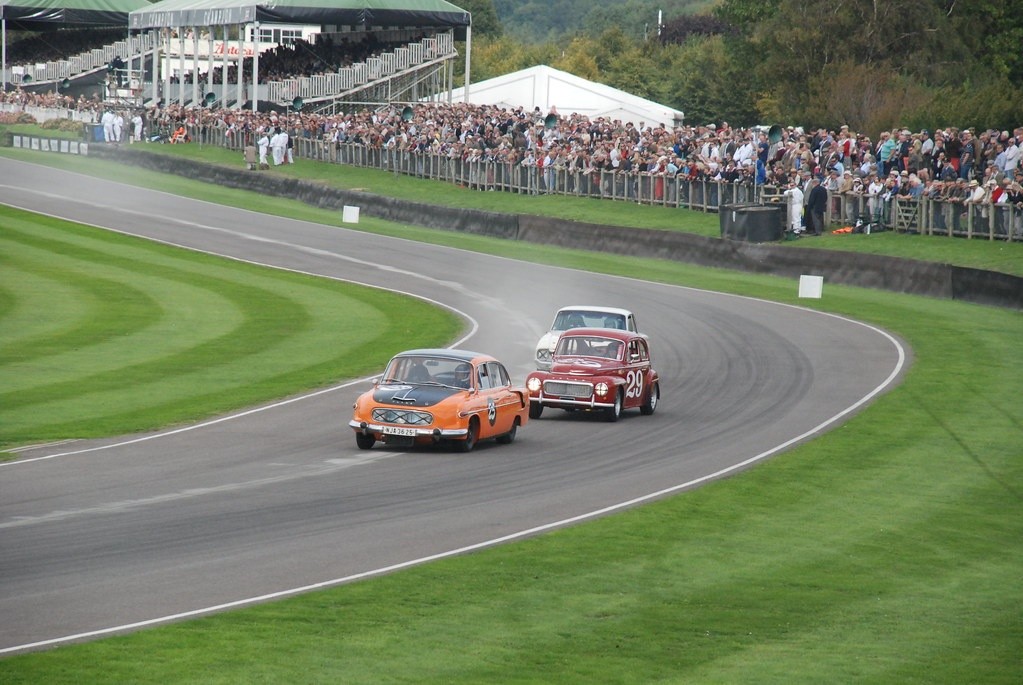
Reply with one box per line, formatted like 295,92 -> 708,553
607,344 -> 618,358
455,364 -> 470,382
603,317 -> 619,328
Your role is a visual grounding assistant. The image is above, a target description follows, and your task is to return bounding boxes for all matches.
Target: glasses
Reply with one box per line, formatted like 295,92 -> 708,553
790,185 -> 794,186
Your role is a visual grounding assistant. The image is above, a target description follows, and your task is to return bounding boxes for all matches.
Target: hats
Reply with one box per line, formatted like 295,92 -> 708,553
890,170 -> 899,175
804,171 -> 812,175
841,125 -> 849,129
853,178 -> 862,183
968,180 -> 979,186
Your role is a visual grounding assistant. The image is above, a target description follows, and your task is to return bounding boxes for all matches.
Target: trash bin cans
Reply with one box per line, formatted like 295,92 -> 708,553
83,122 -> 104,144
717,202 -> 788,244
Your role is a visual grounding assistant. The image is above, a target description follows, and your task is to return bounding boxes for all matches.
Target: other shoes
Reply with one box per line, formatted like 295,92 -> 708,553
794,233 -> 800,238
812,233 -> 821,236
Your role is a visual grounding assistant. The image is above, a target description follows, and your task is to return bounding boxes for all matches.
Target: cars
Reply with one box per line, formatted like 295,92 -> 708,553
525,327 -> 661,422
535,306 -> 649,373
348,349 -> 530,451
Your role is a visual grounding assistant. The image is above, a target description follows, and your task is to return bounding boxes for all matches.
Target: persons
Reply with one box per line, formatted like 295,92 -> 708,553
453,364 -> 481,389
0,83 -> 104,124
604,343 -> 617,359
169,30 -> 445,100
102,55 -> 149,143
752,124 -> 1023,241
603,317 -> 618,329
294,101 -> 753,215
145,104 -> 294,171
162,28 -> 209,41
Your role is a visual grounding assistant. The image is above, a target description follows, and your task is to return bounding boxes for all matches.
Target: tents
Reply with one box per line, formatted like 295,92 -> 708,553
128,0 -> 473,112
0,0 -> 155,92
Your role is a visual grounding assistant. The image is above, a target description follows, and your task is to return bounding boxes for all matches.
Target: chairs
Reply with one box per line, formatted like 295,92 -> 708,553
404,364 -> 431,382
563,314 -> 586,329
